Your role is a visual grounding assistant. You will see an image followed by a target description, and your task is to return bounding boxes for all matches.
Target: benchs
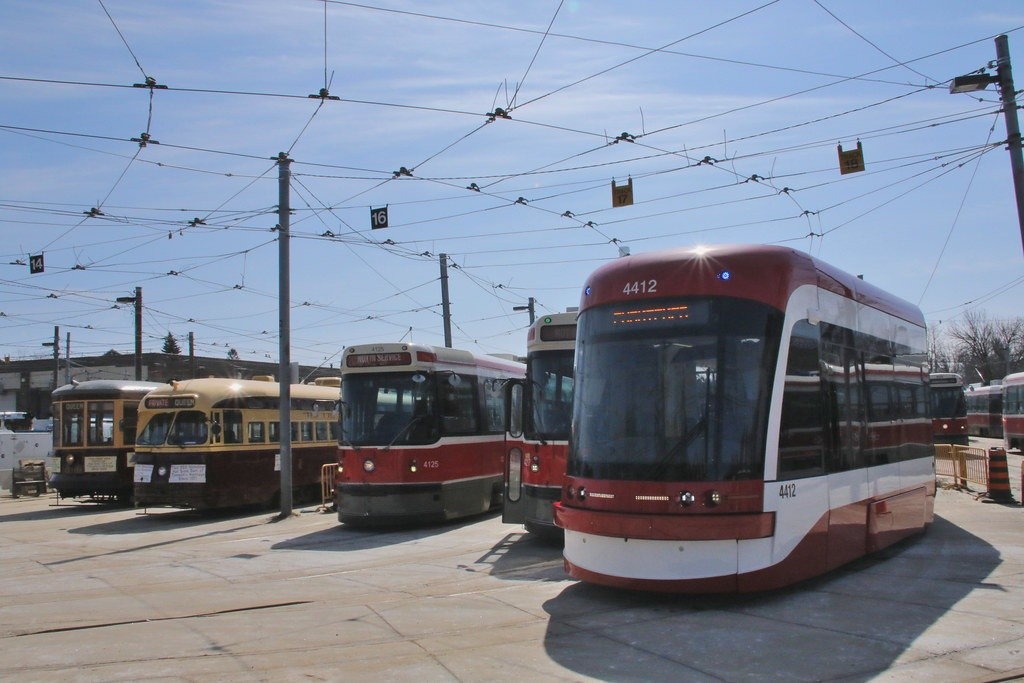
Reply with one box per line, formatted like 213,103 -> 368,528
11,465 -> 47,497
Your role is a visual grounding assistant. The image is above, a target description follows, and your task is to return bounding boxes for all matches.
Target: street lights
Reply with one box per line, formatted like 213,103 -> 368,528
512,297 -> 535,326
116,286 -> 144,381
42,326 -> 59,389
949,35 -> 1024,252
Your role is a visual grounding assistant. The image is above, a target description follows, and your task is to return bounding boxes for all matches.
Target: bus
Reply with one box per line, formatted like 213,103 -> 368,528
330,342 -> 575,528
46,378 -> 170,504
929,372 -> 969,445
1002,371 -> 1024,453
501,304 -> 699,540
132,346 -> 414,511
552,242 -> 938,594
964,385 -> 1004,438
